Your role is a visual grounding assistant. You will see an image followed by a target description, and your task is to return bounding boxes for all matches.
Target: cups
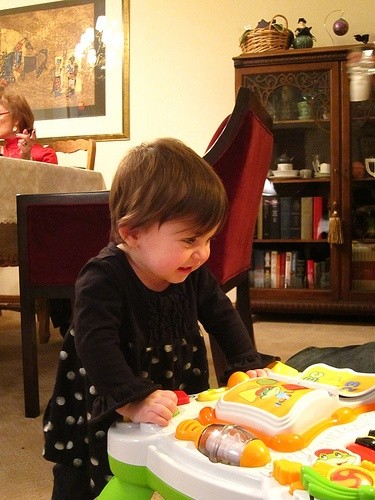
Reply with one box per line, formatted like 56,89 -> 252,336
299,170 -> 312,177
0,138 -> 5,156
297,102 -> 313,116
317,162 -> 330,173
277,163 -> 293,170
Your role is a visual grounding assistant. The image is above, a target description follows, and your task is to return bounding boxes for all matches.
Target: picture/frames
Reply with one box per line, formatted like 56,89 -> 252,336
0,0 -> 130,145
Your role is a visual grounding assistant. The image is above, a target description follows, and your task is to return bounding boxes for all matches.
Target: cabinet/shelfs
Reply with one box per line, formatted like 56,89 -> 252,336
232,43 -> 375,328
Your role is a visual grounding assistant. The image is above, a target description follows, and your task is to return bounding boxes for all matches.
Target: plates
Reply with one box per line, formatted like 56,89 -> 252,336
301,177 -> 312,179
269,176 -> 303,179
314,173 -> 331,178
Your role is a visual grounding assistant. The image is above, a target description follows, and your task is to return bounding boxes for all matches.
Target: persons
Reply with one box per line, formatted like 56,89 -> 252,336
0,93 -> 58,166
40,138 -> 270,500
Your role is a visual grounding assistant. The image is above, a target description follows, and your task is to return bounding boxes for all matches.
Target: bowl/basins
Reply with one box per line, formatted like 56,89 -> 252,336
271,170 -> 299,176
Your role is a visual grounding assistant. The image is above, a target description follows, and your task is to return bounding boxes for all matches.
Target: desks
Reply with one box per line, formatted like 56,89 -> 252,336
0,156 -> 106,417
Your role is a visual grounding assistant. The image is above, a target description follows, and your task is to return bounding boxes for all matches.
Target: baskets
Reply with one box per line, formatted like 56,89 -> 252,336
240,15 -> 294,53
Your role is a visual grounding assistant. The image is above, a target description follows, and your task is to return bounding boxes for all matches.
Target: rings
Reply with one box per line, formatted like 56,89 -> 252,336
22,142 -> 26,146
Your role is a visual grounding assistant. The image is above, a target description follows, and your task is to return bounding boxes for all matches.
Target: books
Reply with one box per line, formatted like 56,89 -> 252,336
248,249 -> 331,291
253,193 -> 323,240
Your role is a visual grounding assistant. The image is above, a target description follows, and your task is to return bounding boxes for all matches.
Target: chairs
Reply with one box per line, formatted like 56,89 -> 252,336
0,139 -> 96,345
16,86 -> 277,418
